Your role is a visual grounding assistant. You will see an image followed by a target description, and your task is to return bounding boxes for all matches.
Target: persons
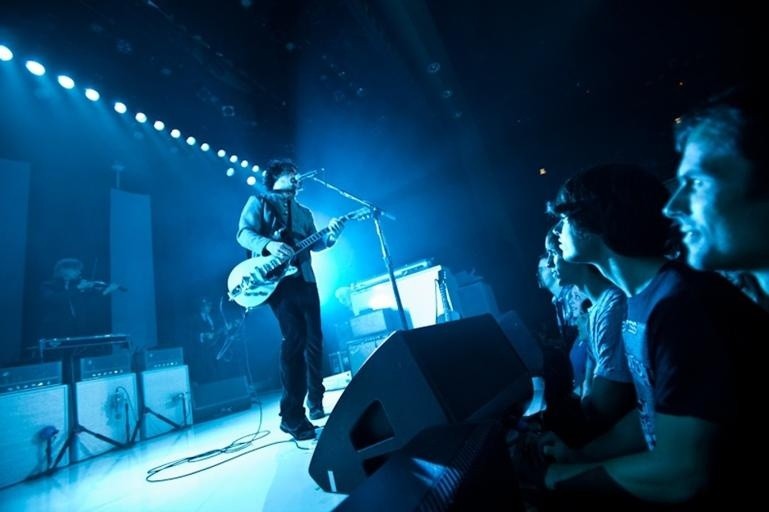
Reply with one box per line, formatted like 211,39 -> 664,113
236,158 -> 343,441
187,295 -> 233,382
35,258 -> 121,360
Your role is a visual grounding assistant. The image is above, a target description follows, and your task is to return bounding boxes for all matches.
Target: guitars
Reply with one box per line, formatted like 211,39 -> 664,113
227,207 -> 375,310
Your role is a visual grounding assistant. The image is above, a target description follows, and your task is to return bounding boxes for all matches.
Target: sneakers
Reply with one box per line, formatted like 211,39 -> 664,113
306,399 -> 324,418
280,417 -> 315,440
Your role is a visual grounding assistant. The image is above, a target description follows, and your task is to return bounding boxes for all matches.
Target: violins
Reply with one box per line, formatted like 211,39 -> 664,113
75,278 -> 130,294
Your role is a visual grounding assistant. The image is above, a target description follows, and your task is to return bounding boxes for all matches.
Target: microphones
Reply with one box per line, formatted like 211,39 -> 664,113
113,389 -> 122,420
39,426 -> 59,441
290,168 -> 325,183
173,392 -> 189,401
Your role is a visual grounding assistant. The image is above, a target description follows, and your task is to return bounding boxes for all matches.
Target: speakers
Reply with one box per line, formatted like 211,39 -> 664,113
345,332 -> 392,380
309,312 -> 533,493
0,383 -> 70,489
137,364 -> 193,439
349,307 -> 395,338
73,371 -> 141,461
